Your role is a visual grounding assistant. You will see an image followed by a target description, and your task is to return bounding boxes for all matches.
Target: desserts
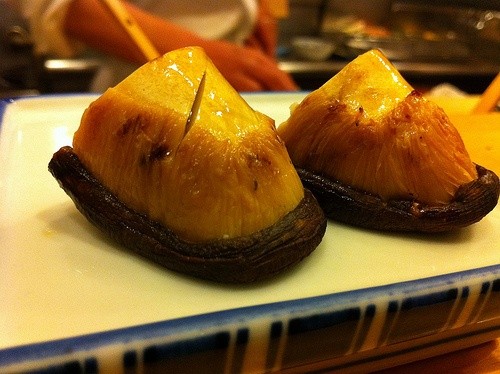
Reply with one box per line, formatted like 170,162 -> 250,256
277,48 -> 500,233
48,45 -> 328,282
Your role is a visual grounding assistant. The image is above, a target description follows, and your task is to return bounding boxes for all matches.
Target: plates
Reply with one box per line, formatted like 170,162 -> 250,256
0,92 -> 500,374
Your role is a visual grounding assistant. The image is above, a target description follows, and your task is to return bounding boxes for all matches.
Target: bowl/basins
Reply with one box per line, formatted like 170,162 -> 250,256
292,39 -> 336,62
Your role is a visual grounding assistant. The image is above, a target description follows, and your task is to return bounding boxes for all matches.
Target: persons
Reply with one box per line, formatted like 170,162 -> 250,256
17,0 -> 301,92
